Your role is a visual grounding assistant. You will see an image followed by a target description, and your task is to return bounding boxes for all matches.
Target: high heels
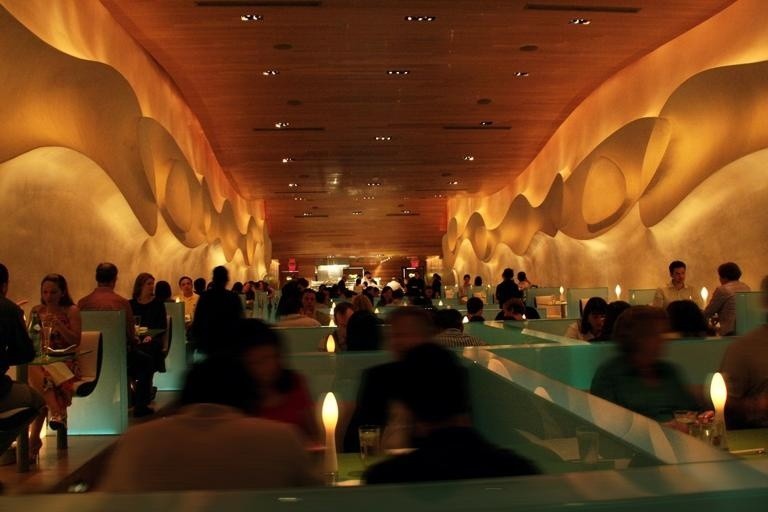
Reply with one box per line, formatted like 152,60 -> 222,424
24,436 -> 43,465
48,418 -> 68,435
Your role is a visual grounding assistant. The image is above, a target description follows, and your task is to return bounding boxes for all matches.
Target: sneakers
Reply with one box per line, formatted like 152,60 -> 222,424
132,404 -> 155,418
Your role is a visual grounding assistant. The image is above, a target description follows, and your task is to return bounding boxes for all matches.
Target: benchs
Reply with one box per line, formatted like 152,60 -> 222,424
126,287 -> 279,390
281,276 -> 767,510
0,308 -> 172,483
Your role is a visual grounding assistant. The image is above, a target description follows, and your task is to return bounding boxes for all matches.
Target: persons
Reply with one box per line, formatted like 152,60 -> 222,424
341,306 -> 470,452
97,355 -> 317,489
237,320 -> 320,446
360,339 -> 539,480
0,264 -> 48,465
719,274 -> 768,430
123,270 -> 165,420
23,271 -> 82,459
591,303 -> 707,424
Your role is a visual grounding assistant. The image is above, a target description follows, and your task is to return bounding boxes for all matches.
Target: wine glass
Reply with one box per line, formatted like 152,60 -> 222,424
42,318 -> 54,359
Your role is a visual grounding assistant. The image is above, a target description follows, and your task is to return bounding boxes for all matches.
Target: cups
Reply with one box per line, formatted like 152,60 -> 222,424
671,406 -> 722,448
324,473 -> 339,487
357,426 -> 380,462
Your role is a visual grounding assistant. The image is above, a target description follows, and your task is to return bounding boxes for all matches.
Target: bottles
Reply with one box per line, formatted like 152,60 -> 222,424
27,311 -> 41,359
